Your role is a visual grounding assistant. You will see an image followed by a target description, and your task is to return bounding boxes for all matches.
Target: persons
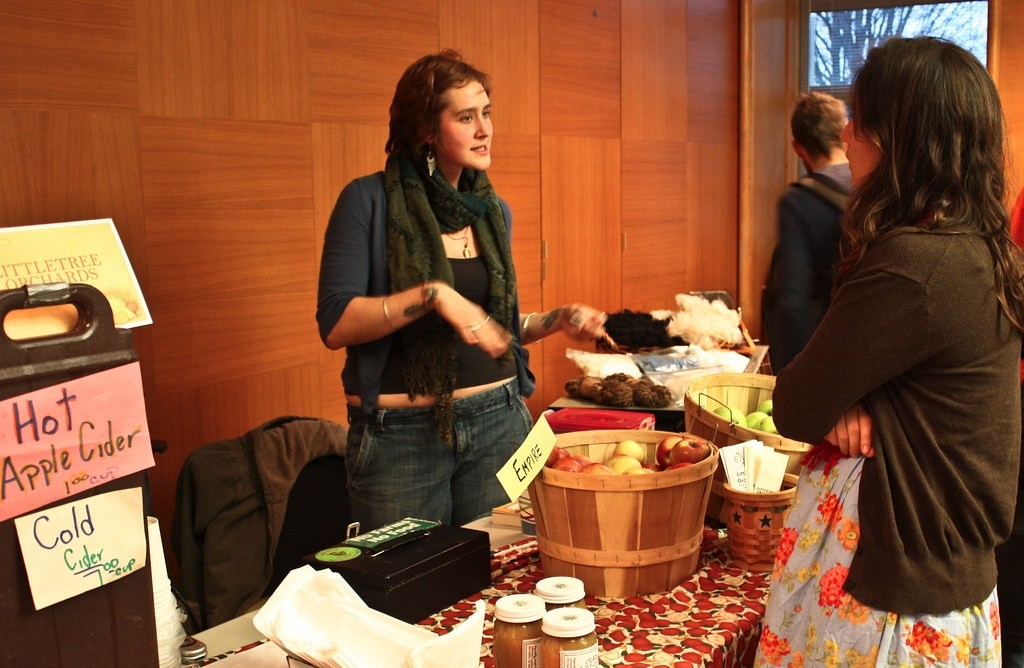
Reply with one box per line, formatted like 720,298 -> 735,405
753,37 -> 1024,668
315,49 -> 608,539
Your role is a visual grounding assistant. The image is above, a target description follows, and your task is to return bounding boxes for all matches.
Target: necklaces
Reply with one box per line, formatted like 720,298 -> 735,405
447,226 -> 471,259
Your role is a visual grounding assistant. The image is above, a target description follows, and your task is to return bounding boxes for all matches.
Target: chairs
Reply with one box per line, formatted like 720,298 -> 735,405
192,416 -> 352,626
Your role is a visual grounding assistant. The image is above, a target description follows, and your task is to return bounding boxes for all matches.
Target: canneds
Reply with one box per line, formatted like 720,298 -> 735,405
491,575 -> 599,668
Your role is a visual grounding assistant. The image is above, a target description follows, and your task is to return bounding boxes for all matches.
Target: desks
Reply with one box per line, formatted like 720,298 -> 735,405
187,486 -> 771,668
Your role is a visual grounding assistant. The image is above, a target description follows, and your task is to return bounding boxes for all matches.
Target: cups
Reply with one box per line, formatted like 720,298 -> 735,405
145,516 -> 183,668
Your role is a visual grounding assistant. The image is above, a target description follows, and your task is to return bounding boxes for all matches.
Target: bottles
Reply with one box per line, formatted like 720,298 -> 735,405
540,607 -> 599,668
493,594 -> 547,668
533,576 -> 586,611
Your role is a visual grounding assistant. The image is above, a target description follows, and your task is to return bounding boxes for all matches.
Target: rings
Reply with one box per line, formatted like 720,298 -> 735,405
469,315 -> 489,331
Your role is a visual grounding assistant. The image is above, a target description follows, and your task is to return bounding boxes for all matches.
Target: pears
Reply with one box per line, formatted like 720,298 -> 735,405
713,399 -> 777,434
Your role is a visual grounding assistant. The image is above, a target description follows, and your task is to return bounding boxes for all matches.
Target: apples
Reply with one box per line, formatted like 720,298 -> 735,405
546,435 -> 710,476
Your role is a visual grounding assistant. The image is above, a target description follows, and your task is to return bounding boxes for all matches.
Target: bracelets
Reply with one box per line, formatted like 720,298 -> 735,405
523,312 -> 542,345
384,297 -> 398,331
422,282 -> 431,312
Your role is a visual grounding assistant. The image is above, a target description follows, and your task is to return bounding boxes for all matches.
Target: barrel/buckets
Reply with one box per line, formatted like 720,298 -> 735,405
528,419 -> 717,598
687,374 -> 808,524
714,475 -> 803,563
